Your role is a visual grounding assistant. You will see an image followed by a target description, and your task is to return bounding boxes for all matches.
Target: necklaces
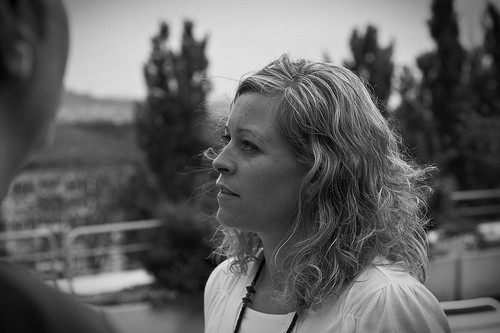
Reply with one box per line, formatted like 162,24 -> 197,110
232,254 -> 306,333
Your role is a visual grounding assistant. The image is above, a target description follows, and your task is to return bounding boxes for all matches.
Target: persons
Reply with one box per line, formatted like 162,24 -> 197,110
203,53 -> 453,333
0,0 -> 122,333
